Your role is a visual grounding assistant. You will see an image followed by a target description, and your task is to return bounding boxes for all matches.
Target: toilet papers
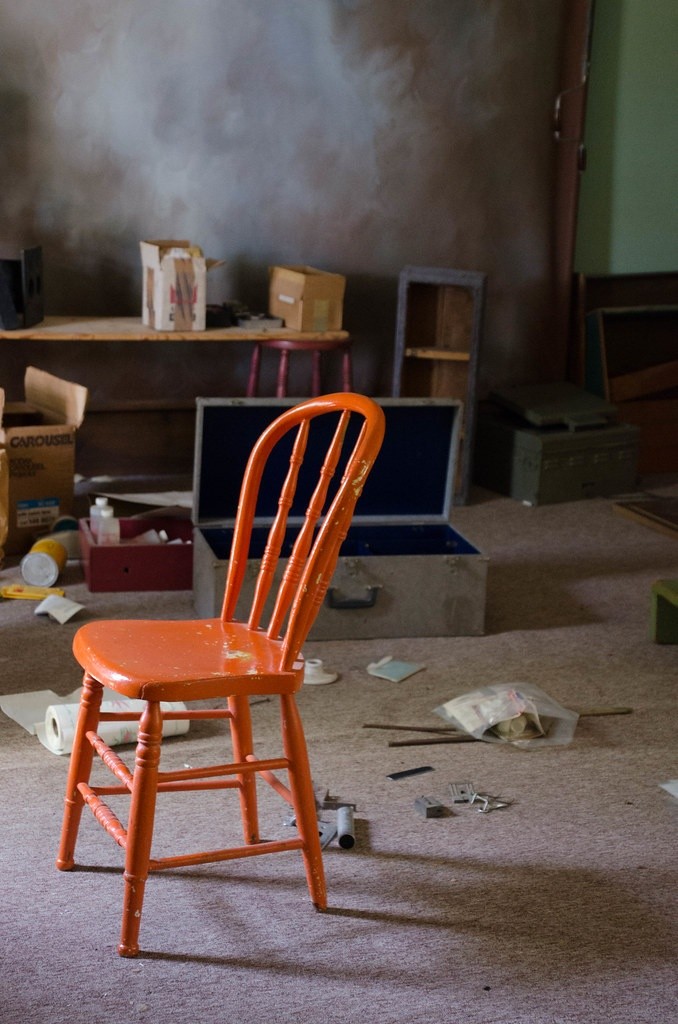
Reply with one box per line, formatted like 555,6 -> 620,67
0,687 -> 190,754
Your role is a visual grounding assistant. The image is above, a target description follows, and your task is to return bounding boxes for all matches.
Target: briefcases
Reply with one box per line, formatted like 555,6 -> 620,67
190,397 -> 491,644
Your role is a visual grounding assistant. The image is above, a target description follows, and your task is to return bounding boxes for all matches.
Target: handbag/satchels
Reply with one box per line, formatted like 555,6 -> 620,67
431,678 -> 581,751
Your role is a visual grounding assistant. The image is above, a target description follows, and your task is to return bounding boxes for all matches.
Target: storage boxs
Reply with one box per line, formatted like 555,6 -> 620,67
77,516 -> 192,594
479,414 -> 645,508
138,238 -> 223,331
268,264 -> 347,333
194,396 -> 487,641
0,365 -> 88,555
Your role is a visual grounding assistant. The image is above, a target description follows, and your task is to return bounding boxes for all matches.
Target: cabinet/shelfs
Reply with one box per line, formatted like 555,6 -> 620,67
0,314 -> 350,498
392,270 -> 489,505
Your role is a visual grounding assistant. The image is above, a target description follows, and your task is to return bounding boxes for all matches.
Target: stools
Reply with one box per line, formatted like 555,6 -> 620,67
247,339 -> 354,399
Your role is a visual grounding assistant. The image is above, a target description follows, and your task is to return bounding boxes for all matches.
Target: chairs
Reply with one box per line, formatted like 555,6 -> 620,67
56,391 -> 386,958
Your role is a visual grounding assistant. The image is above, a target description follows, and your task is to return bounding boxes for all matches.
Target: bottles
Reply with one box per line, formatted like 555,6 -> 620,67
97,507 -> 120,545
122,529 -> 168,546
89,497 -> 110,535
167,537 -> 194,545
